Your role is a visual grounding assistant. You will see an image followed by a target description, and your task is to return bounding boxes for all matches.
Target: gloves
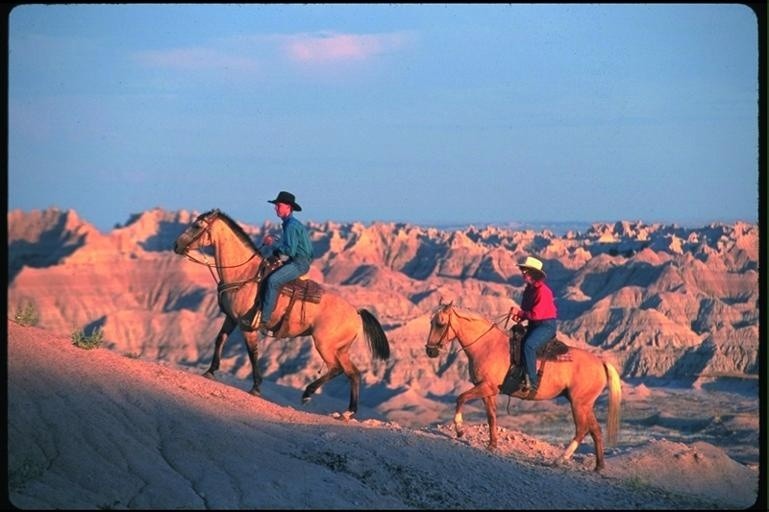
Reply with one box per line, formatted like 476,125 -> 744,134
264,236 -> 272,246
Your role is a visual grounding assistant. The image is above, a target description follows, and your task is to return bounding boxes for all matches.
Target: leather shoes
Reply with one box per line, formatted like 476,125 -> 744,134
240,313 -> 272,328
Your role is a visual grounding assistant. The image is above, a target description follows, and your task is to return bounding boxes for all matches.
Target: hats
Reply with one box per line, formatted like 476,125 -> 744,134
267,191 -> 302,211
515,256 -> 548,279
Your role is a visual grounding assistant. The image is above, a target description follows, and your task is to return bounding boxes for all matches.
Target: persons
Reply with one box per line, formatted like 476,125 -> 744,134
241,192 -> 312,336
509,256 -> 557,392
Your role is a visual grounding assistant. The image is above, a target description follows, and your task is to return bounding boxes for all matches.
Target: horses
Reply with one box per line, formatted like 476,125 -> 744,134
425,294 -> 623,477
173,207 -> 390,421
